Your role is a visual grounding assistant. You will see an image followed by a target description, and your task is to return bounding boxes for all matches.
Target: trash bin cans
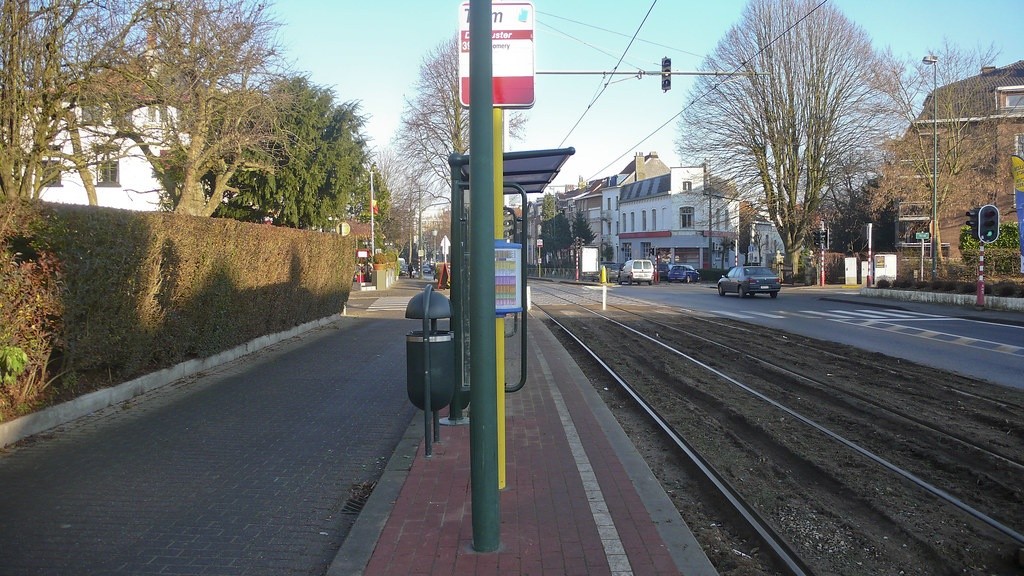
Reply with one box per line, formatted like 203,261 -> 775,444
404,283 -> 455,413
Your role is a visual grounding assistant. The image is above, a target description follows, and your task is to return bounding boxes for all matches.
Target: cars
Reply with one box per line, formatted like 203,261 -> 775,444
605,263 -> 621,282
399,268 -> 409,276
667,265 -> 702,284
716,265 -> 781,299
657,263 -> 674,280
422,265 -> 431,274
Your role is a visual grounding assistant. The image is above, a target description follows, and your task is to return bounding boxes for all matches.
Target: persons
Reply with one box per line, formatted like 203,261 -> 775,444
408,263 -> 413,278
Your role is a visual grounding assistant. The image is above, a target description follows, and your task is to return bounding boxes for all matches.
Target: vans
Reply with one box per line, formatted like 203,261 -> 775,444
616,259 -> 654,286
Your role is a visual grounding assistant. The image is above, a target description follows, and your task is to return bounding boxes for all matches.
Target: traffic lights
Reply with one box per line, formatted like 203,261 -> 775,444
660,56 -> 672,92
813,231 -> 819,246
966,208 -> 979,241
976,205 -> 1000,243
819,229 -> 826,243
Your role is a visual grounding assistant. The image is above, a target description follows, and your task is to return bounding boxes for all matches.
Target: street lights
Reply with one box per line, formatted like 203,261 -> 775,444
923,56 -> 939,291
359,157 -> 377,267
431,227 -> 439,280
